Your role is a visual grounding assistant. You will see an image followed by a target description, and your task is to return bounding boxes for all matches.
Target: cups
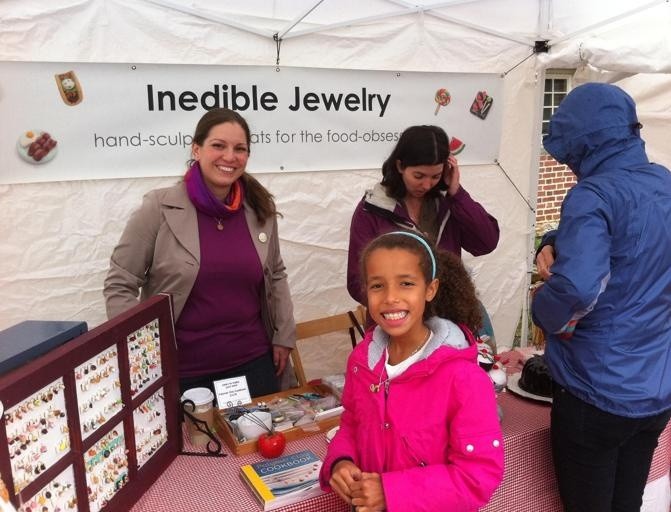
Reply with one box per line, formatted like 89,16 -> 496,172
180,388 -> 215,448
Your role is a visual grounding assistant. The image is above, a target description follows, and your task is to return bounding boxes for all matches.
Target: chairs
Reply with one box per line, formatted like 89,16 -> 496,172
287,306 -> 368,387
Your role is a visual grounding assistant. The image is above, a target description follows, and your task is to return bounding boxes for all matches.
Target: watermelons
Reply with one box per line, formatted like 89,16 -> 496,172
450,136 -> 465,155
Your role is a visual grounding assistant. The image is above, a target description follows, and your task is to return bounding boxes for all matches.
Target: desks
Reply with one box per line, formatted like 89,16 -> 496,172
130,345 -> 671,512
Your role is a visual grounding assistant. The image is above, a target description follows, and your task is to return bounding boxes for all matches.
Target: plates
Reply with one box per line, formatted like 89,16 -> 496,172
16,130 -> 58,164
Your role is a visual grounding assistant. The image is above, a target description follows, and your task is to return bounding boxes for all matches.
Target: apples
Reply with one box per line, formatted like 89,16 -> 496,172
258,428 -> 286,457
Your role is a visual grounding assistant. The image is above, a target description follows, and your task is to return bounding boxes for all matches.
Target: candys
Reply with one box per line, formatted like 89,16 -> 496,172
433,89 -> 451,115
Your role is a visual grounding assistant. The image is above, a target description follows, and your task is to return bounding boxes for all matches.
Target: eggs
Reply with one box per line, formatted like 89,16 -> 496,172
19,130 -> 38,146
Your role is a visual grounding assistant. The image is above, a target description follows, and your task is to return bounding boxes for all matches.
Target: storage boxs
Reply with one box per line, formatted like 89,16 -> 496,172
212,381 -> 345,455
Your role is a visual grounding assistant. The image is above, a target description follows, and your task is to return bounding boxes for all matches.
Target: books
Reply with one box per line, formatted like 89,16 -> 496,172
239,450 -> 322,505
238,473 -> 325,512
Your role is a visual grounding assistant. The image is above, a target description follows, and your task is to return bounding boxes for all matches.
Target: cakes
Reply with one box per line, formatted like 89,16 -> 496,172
518,352 -> 552,399
474,332 -> 507,392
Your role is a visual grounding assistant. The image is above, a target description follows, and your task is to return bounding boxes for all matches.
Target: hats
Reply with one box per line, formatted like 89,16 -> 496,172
506,354 -> 553,405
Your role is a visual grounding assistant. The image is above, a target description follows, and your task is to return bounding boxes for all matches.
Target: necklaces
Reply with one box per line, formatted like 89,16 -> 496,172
372,325 -> 430,393
213,216 -> 224,230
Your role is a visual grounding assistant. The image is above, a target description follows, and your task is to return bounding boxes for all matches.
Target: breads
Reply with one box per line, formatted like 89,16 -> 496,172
28,133 -> 56,160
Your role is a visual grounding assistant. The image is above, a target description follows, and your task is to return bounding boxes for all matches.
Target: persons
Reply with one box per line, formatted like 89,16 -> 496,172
102,106 -> 301,412
529,81 -> 671,512
319,230 -> 506,512
344,124 -> 499,359
